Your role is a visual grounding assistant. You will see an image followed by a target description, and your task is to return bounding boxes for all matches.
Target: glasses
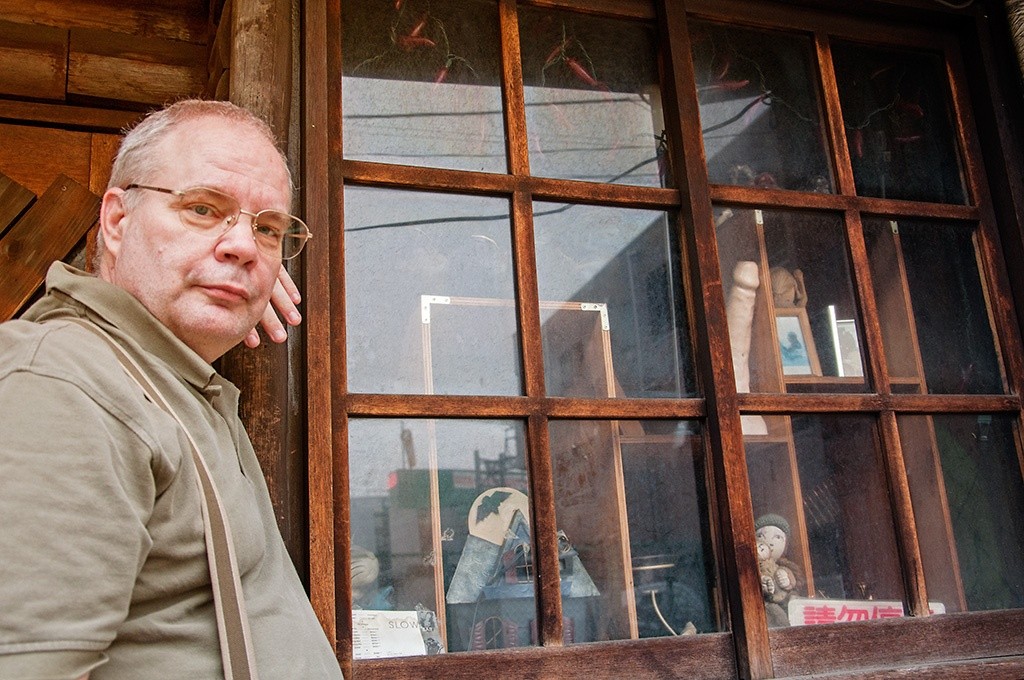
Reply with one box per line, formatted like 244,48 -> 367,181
124,183 -> 313,260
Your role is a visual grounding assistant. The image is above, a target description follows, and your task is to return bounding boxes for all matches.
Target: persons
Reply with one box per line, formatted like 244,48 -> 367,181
0,97 -> 347,680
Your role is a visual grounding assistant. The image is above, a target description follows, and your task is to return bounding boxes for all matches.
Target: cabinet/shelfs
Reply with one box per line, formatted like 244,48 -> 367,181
405,190 -> 967,657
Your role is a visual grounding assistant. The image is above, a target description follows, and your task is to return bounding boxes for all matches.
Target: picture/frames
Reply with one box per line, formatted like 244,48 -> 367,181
775,308 -> 823,378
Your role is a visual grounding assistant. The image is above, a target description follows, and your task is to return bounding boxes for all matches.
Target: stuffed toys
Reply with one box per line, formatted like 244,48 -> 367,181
753,515 -> 804,629
